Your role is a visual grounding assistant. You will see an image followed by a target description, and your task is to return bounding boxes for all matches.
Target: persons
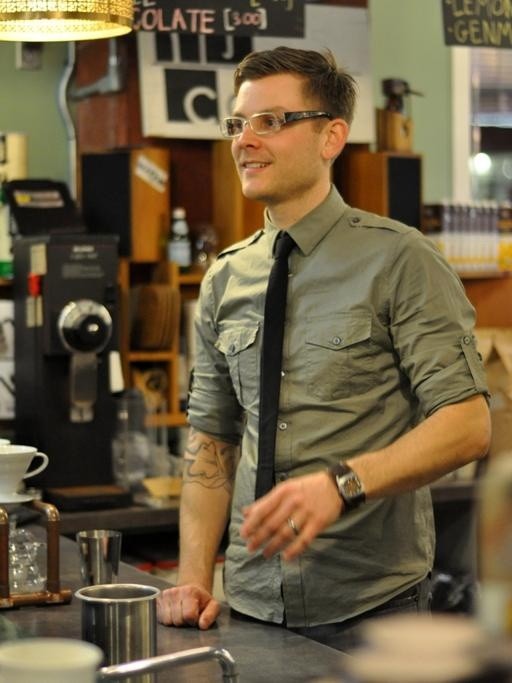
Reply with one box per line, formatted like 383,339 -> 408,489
154,46 -> 492,653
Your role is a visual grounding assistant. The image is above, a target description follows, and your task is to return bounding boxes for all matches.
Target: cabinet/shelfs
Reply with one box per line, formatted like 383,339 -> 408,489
84,139 -> 511,490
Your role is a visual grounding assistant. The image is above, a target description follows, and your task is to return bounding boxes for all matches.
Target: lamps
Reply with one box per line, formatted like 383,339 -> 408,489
0,0 -> 135,42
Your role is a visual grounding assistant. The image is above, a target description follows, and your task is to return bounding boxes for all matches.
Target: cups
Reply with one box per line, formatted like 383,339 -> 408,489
0,638 -> 103,682
75,529 -> 122,585
0,444 -> 49,497
73,582 -> 160,682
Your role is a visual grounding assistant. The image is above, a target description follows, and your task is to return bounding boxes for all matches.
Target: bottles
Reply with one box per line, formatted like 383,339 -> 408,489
165,206 -> 193,273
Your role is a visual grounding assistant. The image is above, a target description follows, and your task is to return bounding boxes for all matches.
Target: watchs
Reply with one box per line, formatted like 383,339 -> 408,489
329,461 -> 366,516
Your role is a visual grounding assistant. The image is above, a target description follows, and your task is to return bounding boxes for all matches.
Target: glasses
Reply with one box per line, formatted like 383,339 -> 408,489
219,109 -> 333,140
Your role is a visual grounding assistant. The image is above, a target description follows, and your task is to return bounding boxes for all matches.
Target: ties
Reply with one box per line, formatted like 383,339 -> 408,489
254,232 -> 296,501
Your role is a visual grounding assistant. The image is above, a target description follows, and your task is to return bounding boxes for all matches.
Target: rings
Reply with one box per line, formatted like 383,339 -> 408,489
286,518 -> 300,536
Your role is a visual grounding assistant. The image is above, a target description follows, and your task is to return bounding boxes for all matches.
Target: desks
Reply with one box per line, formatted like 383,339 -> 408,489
1,481 -> 473,683
